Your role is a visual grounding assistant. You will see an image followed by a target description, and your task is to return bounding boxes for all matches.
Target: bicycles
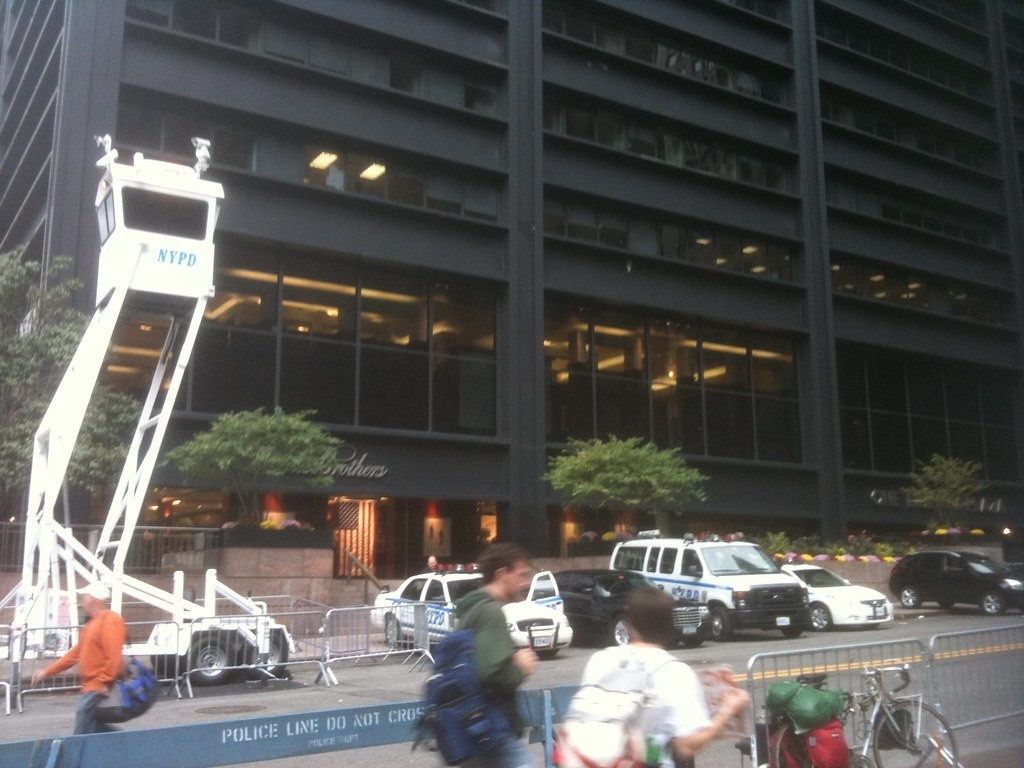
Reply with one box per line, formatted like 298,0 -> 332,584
733,659 -> 959,768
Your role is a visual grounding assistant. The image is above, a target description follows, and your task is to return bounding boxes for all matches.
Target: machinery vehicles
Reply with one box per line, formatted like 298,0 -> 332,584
1,123 -> 294,680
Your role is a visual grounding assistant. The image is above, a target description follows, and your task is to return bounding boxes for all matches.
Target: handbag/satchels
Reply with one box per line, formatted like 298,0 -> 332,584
96,653 -> 163,723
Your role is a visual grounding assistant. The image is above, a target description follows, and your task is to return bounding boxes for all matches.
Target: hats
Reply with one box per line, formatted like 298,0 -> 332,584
76,582 -> 110,602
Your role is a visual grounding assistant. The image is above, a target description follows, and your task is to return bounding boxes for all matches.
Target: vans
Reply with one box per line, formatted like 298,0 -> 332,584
612,529 -> 813,638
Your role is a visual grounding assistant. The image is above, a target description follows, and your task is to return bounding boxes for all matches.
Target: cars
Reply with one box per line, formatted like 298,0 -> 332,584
367,558 -> 575,659
777,564 -> 895,631
520,567 -> 716,657
886,550 -> 1023,617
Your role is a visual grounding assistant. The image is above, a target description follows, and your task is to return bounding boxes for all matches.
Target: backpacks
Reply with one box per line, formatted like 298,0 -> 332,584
554,654 -> 686,768
414,595 -> 519,766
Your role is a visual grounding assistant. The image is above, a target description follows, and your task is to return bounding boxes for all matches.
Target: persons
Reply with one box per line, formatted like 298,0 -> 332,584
31,580 -> 129,735
421,556 -> 437,574
454,542 -> 538,768
578,586 -> 750,766
